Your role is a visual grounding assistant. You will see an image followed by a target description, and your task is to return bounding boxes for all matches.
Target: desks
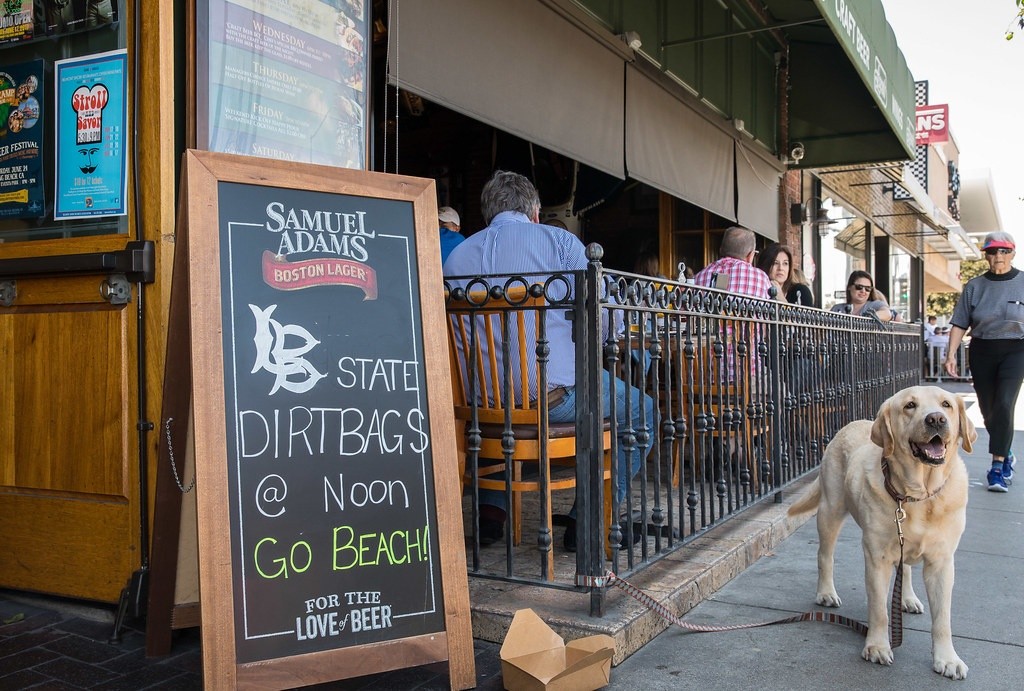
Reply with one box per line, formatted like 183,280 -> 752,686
552,334 -> 733,539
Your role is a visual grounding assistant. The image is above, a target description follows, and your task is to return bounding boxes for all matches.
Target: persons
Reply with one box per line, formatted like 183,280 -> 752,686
441,171 -> 662,550
924,316 -> 973,382
753,244 -> 819,404
942,231 -> 1024,492
694,226 -> 791,477
438,206 -> 466,269
827,269 -> 903,384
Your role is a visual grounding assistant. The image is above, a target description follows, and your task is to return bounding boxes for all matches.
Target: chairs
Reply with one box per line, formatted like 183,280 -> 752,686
444,282 -> 613,582
672,309 -> 759,494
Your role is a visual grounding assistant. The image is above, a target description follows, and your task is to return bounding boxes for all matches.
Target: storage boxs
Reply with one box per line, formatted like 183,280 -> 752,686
498,608 -> 617,691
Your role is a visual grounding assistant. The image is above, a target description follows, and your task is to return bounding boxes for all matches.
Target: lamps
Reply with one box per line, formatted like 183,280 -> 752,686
791,196 -> 838,236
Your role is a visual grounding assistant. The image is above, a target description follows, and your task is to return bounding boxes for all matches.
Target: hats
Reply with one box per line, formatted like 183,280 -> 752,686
981,232 -> 1015,250
438,207 -> 461,226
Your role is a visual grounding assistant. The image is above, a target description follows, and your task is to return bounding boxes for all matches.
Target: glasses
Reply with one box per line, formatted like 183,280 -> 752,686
852,283 -> 873,292
753,250 -> 760,255
986,247 -> 1012,255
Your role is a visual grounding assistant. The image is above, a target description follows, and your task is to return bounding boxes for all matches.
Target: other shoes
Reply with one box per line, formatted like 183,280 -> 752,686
700,442 -> 730,482
473,523 -> 504,547
563,523 -> 641,552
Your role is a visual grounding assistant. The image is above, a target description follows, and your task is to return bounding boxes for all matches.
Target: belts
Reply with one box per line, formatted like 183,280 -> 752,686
516,387 -> 566,410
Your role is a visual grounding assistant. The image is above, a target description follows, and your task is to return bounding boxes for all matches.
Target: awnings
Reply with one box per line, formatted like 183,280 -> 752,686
831,220 -> 867,260
818,161 -> 934,218
872,201 -> 967,261
570,0 -> 918,171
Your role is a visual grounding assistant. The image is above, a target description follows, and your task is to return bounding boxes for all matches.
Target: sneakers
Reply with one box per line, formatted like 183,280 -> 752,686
987,468 -> 1008,492
1002,451 -> 1016,480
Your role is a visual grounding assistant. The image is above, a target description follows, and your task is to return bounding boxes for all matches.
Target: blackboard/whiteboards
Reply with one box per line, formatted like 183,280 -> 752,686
146,149 -> 469,691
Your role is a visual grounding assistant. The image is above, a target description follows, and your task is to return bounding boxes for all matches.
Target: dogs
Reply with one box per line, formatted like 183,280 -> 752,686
788,383 -> 979,682
